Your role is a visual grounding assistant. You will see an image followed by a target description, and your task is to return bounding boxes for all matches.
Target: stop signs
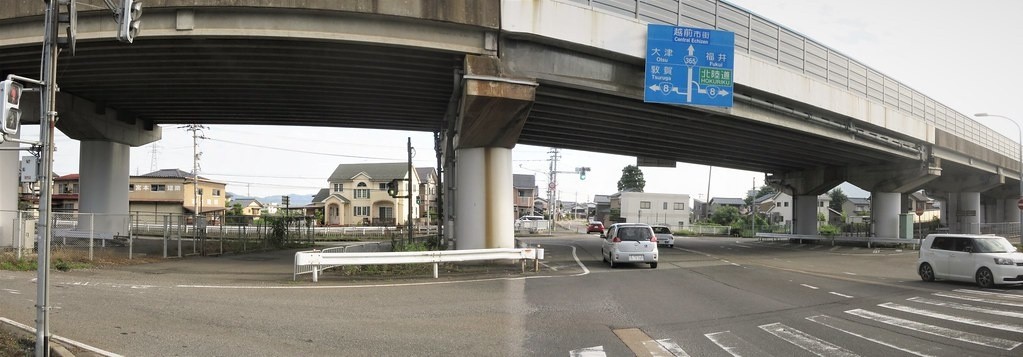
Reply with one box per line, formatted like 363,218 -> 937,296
1017,199 -> 1023,210
915,208 -> 923,216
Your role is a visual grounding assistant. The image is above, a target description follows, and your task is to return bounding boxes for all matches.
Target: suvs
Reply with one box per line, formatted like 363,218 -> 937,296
599,222 -> 659,269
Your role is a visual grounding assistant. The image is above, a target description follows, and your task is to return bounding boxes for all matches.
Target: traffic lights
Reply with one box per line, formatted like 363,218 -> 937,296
416,196 -> 421,204
117,0 -> 144,44
580,167 -> 586,180
386,181 -> 399,196
0,79 -> 24,135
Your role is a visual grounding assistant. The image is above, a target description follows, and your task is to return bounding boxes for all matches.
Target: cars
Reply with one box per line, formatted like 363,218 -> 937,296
585,221 -> 606,234
650,225 -> 674,248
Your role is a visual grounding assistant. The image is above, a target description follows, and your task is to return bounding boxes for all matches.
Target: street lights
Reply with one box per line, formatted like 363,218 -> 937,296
974,112 -> 1023,247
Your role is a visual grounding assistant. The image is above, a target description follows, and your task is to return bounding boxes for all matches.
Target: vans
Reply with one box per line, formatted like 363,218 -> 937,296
916,231 -> 1023,289
515,215 -> 545,228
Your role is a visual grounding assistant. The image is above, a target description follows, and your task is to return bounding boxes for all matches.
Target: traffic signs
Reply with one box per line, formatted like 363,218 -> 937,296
643,23 -> 735,109
